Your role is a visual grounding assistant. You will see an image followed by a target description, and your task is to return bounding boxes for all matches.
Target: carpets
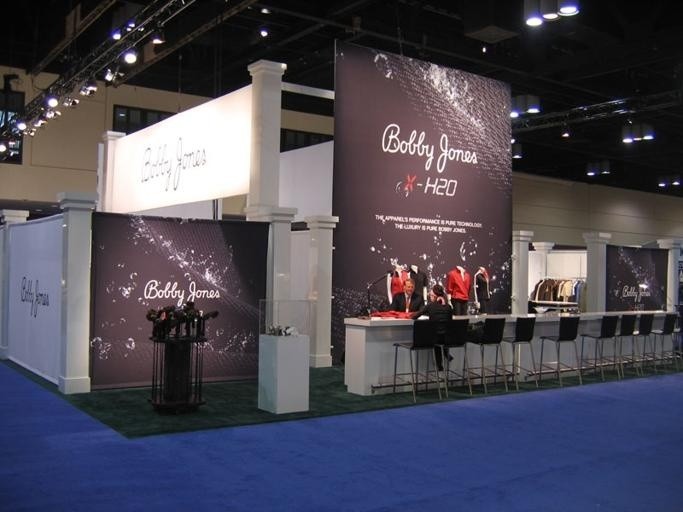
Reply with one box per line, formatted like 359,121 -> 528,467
1,359 -> 681,440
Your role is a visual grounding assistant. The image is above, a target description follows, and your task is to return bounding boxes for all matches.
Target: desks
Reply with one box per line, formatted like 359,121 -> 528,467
343,308 -> 679,402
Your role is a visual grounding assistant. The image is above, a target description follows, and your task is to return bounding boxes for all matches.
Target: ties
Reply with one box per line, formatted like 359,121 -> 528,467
406,297 -> 409,308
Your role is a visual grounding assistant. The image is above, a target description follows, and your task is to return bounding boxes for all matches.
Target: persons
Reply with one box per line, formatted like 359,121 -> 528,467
447,265 -> 471,315
406,265 -> 428,305
473,266 -> 491,315
386,265 -> 410,305
391,279 -> 424,314
413,285 -> 454,371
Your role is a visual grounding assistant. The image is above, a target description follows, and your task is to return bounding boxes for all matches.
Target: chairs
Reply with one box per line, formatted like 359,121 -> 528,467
389,314 -> 681,402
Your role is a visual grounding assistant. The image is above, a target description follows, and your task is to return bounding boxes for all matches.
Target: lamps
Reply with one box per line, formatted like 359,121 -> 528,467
509,90 -> 656,177
524,0 -> 579,29
